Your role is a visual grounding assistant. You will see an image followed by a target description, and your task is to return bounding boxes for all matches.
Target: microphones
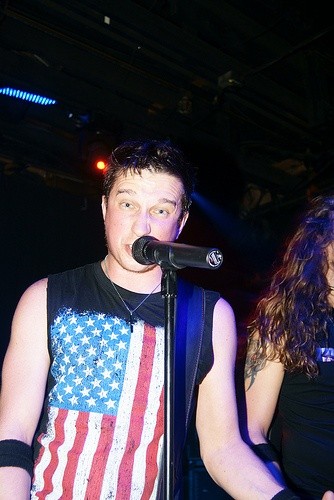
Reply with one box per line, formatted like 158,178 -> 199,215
132,236 -> 223,271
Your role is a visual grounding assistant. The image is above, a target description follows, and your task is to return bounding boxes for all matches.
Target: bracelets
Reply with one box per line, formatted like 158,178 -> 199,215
271,488 -> 300,500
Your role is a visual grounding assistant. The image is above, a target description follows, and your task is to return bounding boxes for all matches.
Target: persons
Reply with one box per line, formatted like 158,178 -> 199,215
0,140 -> 300,500
244,195 -> 334,500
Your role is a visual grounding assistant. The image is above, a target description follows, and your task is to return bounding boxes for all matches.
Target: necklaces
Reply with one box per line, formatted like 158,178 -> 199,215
104,254 -> 161,316
